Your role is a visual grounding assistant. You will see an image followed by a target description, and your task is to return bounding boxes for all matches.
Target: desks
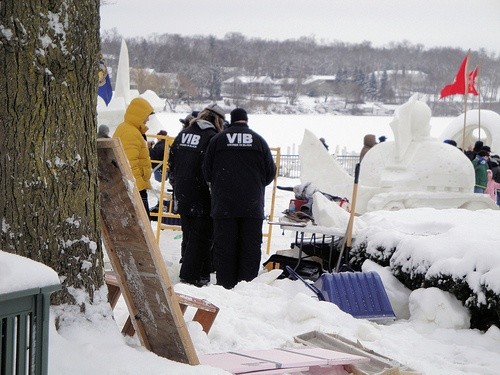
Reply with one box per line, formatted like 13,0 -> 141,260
279,225 -> 356,281
147,170 -> 181,226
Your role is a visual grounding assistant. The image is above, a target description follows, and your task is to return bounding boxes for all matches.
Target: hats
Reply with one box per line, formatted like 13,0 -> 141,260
179,114 -> 193,124
157,130 -> 167,136
205,104 -> 226,118
379,136 -> 387,140
150,112 -> 155,114
481,146 -> 491,152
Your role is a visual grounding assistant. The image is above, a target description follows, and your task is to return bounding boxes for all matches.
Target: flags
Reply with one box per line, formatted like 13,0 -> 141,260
96,58 -> 113,106
440,49 -> 469,100
466,64 -> 479,96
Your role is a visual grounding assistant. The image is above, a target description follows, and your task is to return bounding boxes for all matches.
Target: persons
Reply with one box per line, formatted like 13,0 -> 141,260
148,130 -> 174,183
378,136 -> 387,143
200,107 -> 278,290
319,138 -> 330,151
167,100 -> 226,288
97,125 -> 111,139
112,98 -> 156,229
443,139 -> 500,208
359,134 -> 378,164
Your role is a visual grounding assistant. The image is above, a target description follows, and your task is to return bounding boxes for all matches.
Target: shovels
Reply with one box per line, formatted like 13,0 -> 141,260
285,264 -> 396,322
